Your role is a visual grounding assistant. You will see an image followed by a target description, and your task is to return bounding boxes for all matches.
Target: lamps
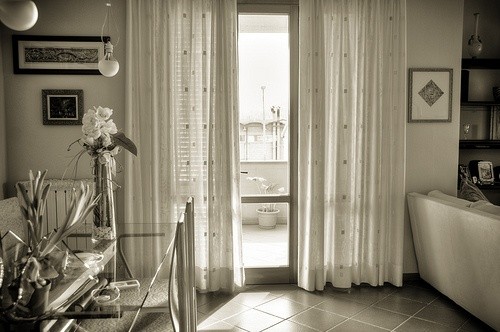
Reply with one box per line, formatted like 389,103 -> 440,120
0,0 -> 39,31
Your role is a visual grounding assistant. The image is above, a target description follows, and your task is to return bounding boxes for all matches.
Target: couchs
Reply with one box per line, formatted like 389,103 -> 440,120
407,190 -> 500,332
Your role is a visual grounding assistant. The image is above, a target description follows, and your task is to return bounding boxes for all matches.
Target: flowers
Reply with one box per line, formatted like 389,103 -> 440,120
61,106 -> 137,229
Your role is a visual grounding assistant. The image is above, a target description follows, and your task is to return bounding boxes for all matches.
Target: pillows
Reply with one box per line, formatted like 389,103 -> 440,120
458,178 -> 489,202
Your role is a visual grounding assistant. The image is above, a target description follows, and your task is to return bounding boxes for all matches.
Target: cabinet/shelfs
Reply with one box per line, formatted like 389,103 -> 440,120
458,58 -> 500,203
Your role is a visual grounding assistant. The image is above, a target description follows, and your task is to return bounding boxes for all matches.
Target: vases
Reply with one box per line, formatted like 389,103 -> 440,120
92,158 -> 117,238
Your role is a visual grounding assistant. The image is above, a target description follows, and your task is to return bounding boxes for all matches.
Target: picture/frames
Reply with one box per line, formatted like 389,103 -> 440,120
478,161 -> 495,185
12,34 -> 111,76
42,89 -> 85,126
408,67 -> 453,123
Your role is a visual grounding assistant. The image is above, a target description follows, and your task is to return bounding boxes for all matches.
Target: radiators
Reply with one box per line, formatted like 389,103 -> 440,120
17,179 -> 97,252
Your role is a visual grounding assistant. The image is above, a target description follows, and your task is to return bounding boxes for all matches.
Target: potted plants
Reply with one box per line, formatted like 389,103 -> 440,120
7,169 -> 102,318
247,177 -> 284,229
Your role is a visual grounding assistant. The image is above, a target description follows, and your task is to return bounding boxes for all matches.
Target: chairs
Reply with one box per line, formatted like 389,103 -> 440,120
71,197 -> 197,332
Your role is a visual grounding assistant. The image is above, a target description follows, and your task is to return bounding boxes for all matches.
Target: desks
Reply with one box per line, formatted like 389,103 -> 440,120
0,222 -> 178,332
